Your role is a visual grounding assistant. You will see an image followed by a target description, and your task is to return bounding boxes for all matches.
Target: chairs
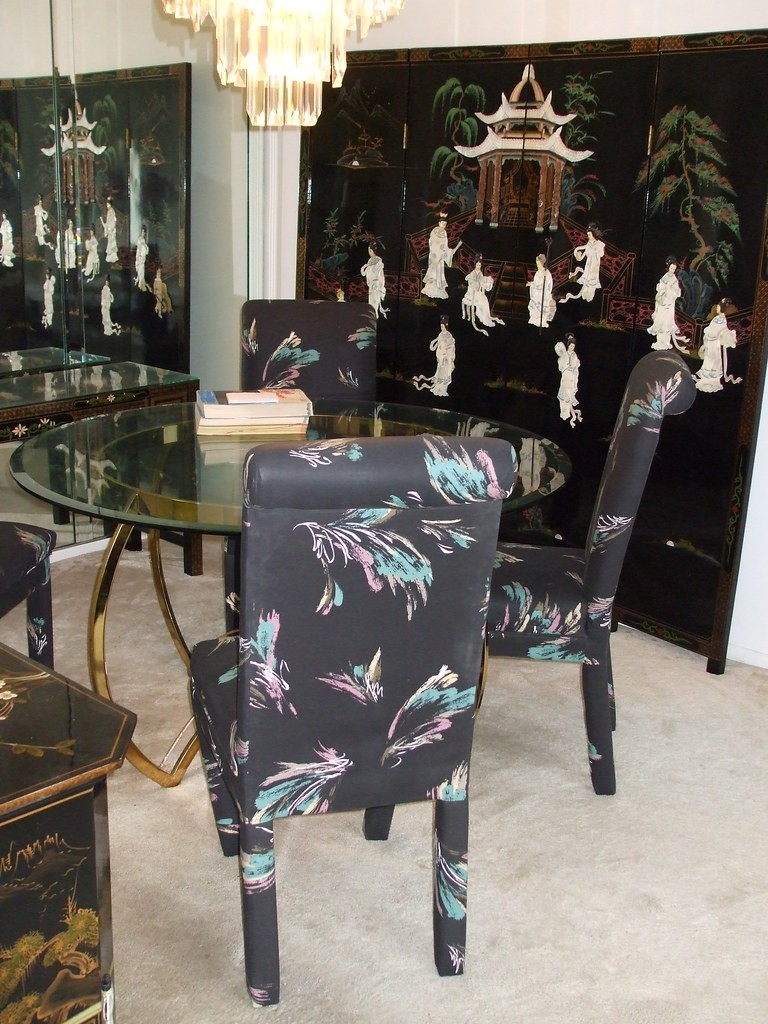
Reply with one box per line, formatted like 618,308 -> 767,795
188,436 -> 519,1010
224,298 -> 374,638
486,349 -> 695,796
0,520 -> 59,675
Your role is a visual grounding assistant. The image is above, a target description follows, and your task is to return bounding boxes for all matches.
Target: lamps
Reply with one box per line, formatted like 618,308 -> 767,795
162,0 -> 407,129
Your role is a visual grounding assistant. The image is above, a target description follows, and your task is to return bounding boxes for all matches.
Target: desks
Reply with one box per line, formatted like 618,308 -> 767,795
0,347 -> 114,524
0,359 -> 203,576
9,395 -> 570,791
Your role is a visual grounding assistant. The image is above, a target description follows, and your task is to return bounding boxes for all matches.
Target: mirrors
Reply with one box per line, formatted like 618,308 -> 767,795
0,0 -> 252,558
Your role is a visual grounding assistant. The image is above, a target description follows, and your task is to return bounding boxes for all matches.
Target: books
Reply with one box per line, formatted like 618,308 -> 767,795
194,389 -> 313,435
195,435 -> 309,465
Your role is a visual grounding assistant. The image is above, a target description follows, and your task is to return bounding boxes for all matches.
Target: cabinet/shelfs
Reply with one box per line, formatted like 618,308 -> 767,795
0,641 -> 142,1024
